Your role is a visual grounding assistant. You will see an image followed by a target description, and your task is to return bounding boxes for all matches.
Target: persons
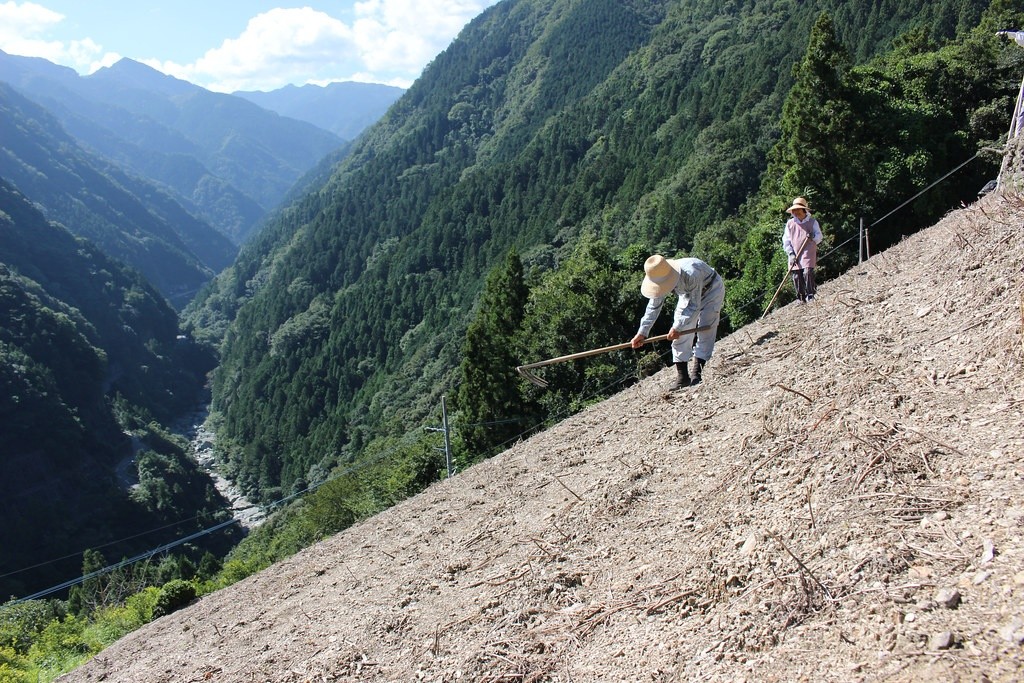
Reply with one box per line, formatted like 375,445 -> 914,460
630,255 -> 727,394
781,195 -> 823,305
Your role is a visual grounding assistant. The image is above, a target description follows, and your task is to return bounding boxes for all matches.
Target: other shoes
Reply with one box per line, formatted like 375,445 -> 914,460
808,294 -> 814,302
798,297 -> 806,305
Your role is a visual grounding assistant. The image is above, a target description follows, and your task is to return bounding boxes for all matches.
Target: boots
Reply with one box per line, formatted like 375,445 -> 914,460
669,361 -> 691,391
690,356 -> 706,387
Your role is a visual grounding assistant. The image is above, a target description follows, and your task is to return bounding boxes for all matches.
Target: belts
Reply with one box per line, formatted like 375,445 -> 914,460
702,271 -> 718,292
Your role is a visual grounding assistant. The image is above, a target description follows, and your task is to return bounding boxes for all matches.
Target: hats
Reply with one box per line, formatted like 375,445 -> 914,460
786,198 -> 810,213
641,254 -> 681,299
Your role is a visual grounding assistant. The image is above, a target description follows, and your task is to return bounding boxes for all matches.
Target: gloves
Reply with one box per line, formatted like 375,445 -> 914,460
805,230 -> 815,240
789,252 -> 798,266
